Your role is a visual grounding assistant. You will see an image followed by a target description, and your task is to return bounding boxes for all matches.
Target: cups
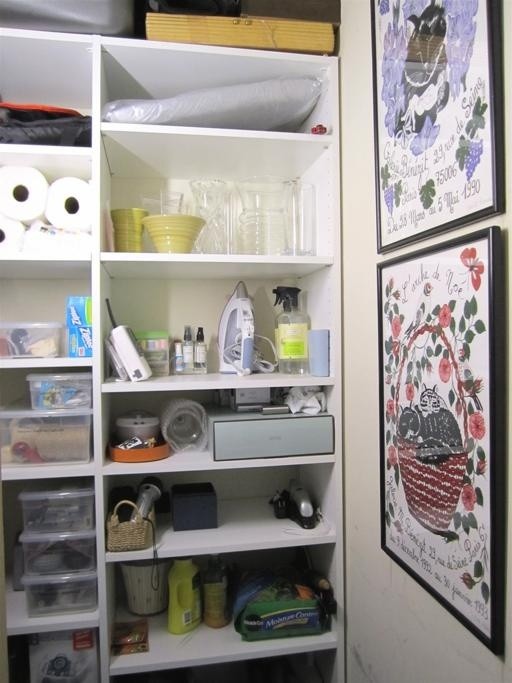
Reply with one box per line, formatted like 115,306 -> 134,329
111,206 -> 149,253
186,175 -> 231,253
233,173 -> 293,253
292,182 -> 318,256
159,190 -> 184,214
307,328 -> 329,376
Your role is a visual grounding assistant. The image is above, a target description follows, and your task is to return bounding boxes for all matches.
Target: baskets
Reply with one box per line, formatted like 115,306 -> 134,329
106,498 -> 157,551
10,417 -> 88,459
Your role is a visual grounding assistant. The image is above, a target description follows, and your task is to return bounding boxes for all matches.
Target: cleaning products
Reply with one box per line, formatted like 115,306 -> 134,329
271,286 -> 310,375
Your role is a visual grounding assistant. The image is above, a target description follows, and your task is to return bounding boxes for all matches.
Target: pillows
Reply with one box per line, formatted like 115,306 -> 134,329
99,75 -> 324,129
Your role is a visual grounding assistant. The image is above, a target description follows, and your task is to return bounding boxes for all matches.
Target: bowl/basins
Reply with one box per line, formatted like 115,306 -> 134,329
109,438 -> 170,462
139,212 -> 208,253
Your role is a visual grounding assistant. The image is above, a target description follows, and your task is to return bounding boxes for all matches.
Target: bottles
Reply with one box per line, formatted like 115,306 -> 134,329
173,339 -> 185,372
303,569 -> 333,591
183,325 -> 195,371
194,325 -> 208,374
200,553 -> 233,629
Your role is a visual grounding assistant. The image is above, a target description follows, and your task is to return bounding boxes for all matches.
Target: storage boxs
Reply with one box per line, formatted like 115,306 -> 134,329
229,573 -> 322,641
0,321 -> 99,682
97,33 -> 347,682
168,479 -> 219,531
132,330 -> 171,376
194,397 -> 335,461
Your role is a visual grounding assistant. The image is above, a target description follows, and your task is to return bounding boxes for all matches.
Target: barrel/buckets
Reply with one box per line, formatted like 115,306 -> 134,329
166,558 -> 202,635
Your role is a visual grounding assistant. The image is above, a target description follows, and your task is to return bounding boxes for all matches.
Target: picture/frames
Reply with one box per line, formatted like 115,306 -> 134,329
369,0 -> 507,253
374,222 -> 510,666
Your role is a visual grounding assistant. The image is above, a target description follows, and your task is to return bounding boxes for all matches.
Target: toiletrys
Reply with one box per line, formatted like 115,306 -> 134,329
174,324 -> 208,375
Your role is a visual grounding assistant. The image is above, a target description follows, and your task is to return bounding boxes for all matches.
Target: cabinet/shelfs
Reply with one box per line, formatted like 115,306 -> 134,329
0,26 -> 105,682
